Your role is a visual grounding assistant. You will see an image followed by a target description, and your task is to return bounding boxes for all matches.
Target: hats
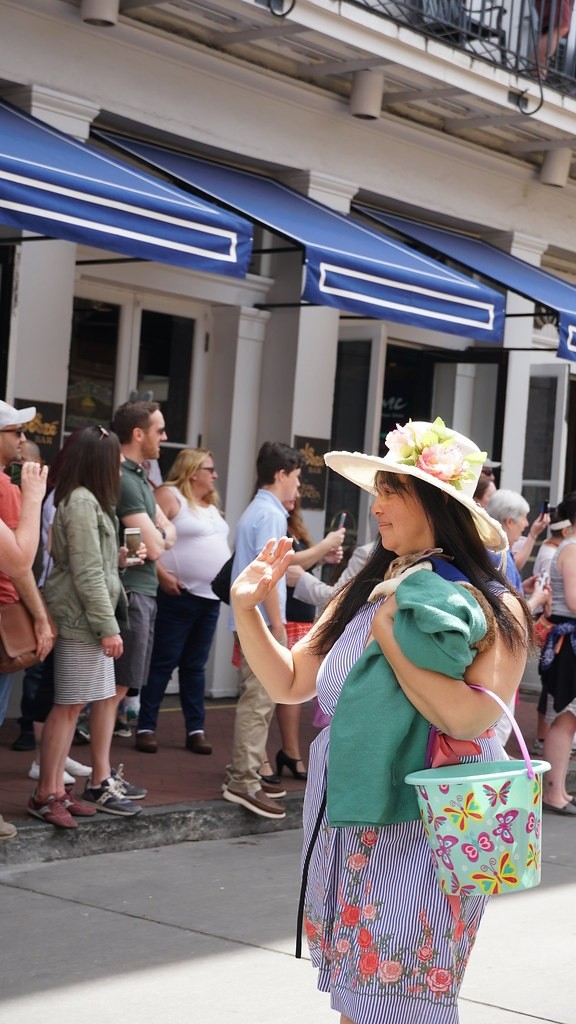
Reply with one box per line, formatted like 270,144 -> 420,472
0,400 -> 36,427
322,416 -> 510,556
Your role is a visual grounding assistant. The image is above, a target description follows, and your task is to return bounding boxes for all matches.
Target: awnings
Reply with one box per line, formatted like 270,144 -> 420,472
89,124 -> 505,346
0,95 -> 255,281
351,203 -> 576,361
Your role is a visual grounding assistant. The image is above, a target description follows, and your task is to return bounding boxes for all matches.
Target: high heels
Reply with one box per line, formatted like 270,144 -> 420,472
276,749 -> 307,780
256,760 -> 281,784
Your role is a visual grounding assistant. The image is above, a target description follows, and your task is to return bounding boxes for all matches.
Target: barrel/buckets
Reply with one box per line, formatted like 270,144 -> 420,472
404,684 -> 551,897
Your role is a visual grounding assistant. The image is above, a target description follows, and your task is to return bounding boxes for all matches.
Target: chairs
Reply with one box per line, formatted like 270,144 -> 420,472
412,0 -> 508,69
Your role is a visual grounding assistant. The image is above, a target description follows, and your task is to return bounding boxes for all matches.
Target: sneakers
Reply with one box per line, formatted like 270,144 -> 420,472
26,756 -> 148,828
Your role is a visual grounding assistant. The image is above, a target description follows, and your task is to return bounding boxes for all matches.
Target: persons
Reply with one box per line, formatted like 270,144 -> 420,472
532,0 -> 574,78
222,439 -> 304,824
26,390 -> 176,826
0,462 -> 58,842
468,456 -> 576,819
0,390 -> 351,755
229,415 -> 530,1024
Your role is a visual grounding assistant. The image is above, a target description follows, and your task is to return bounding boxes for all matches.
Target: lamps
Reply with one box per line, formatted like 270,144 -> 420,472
349,69 -> 383,120
81,0 -> 120,28
541,148 -> 571,188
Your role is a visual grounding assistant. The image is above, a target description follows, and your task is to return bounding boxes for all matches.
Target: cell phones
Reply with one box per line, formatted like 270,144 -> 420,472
9,462 -> 23,486
338,512 -> 347,529
124,528 -> 141,563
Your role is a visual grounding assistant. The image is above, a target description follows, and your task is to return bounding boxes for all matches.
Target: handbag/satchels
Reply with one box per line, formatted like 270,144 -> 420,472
0,591 -> 58,674
210,551 -> 232,605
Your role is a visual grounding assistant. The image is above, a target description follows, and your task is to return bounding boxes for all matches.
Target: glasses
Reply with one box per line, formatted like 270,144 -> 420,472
198,468 -> 216,474
94,424 -> 110,440
0,427 -> 23,437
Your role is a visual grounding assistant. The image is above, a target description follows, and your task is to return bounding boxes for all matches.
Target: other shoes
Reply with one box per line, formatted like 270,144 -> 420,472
526,735 -> 576,818
185,733 -> 211,755
0,815 -> 18,837
136,729 -> 158,754
222,772 -> 286,819
113,718 -> 132,738
11,717 -> 36,751
71,730 -> 90,746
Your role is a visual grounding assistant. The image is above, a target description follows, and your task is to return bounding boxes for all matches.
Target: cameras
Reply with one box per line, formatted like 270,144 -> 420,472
541,500 -> 557,515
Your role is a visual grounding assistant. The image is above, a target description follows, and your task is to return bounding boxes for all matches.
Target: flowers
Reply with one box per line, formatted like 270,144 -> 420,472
384,417 -> 490,490
